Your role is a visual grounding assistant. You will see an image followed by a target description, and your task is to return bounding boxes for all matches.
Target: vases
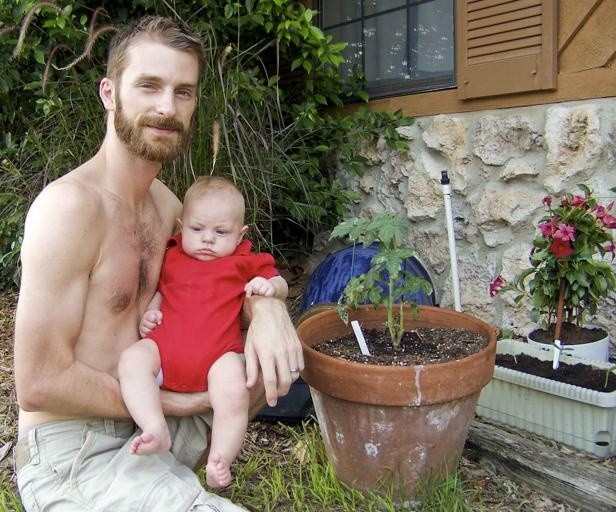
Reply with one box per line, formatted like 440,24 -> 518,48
476,321 -> 616,460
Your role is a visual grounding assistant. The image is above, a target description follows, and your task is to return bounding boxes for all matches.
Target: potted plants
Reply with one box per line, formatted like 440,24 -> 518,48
295,208 -> 496,503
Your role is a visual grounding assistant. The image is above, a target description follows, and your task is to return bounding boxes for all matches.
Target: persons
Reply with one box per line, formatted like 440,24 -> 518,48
115,176 -> 291,490
12,14 -> 307,511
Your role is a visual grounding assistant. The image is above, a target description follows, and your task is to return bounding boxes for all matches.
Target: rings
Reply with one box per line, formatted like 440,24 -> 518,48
289,369 -> 299,373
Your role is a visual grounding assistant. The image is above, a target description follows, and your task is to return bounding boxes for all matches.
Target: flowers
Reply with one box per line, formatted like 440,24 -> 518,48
490,183 -> 616,377
